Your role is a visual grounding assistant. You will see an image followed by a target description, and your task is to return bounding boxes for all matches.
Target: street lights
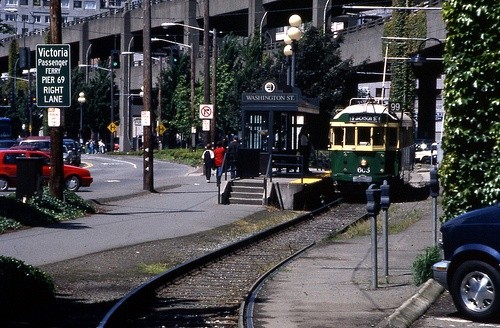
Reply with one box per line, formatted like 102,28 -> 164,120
78,56 -> 114,152
78,91 -> 87,137
283,14 -> 302,150
161,22 -> 217,147
150,37 -> 196,152
121,51 -> 163,136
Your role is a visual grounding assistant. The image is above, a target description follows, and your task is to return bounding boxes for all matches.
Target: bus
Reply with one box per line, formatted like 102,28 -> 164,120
326,42 -> 417,194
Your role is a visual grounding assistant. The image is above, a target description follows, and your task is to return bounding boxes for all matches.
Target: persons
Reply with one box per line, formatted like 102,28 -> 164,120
300,129 -> 308,156
85,138 -> 105,155
201,135 -> 240,182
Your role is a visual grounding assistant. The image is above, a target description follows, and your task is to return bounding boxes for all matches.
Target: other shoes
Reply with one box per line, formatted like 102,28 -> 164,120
206,180 -> 210,183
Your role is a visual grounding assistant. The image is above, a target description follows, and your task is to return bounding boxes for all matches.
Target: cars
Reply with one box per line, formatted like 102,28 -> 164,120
0,137 -> 94,192
413,142 -> 437,165
431,200 -> 500,322
